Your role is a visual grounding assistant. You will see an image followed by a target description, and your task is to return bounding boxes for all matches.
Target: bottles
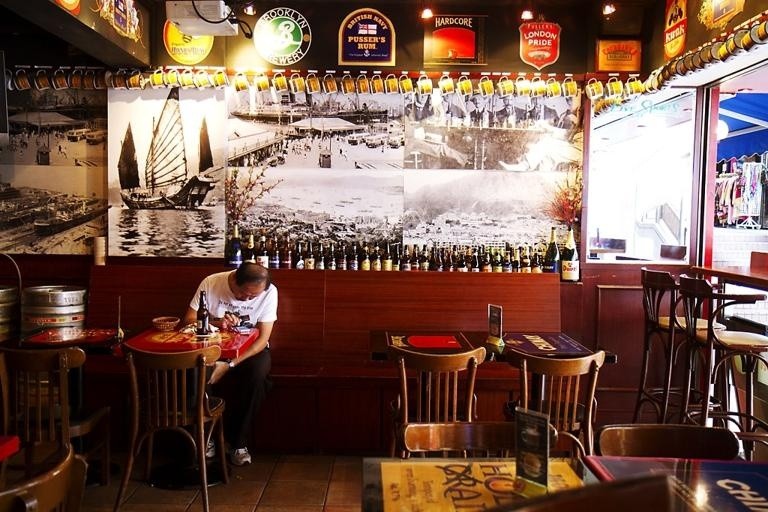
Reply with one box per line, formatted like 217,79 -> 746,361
542,226 -> 561,272
228,223 -> 430,270
560,224 -> 580,282
431,240 -> 543,273
197,290 -> 210,333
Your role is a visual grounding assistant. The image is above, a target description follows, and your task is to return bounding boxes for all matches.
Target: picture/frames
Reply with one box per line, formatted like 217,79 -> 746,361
595,35 -> 644,75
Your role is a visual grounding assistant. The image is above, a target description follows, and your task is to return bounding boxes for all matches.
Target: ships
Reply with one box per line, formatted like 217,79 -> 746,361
34,200 -> 107,236
0,183 -> 98,228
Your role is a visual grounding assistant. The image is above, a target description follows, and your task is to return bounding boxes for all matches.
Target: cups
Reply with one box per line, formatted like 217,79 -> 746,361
458,76 -> 577,98
585,78 -> 644,100
151,70 -> 321,94
645,20 -> 768,94
5,69 -> 145,91
322,74 -> 454,95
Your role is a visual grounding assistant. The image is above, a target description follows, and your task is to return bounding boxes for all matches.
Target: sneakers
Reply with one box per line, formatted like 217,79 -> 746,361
230,446 -> 253,467
204,438 -> 216,458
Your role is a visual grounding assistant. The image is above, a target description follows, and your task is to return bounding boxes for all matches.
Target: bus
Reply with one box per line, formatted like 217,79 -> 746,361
348,133 -> 370,145
366,135 -> 404,149
68,128 -> 90,142
86,130 -> 108,145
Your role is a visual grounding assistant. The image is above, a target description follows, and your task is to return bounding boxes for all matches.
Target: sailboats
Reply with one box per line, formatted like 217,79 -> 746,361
117,87 -> 224,210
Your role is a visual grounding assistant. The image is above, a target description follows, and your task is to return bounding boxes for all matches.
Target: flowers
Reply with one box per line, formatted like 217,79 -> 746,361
225,142 -> 283,223
538,166 -> 584,226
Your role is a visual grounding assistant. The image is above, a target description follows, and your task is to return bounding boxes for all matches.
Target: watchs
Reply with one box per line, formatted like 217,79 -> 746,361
225,359 -> 235,369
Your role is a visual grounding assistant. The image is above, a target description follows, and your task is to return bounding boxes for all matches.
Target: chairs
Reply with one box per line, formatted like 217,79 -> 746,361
677,274 -> 768,463
0,323 -> 128,512
633,266 -> 728,429
114,344 -> 230,512
597,424 -> 739,460
385,345 -> 487,458
503,349 -> 605,456
404,422 -> 558,458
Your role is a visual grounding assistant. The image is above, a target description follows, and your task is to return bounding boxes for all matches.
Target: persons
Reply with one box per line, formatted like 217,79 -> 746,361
183,262 -> 279,467
